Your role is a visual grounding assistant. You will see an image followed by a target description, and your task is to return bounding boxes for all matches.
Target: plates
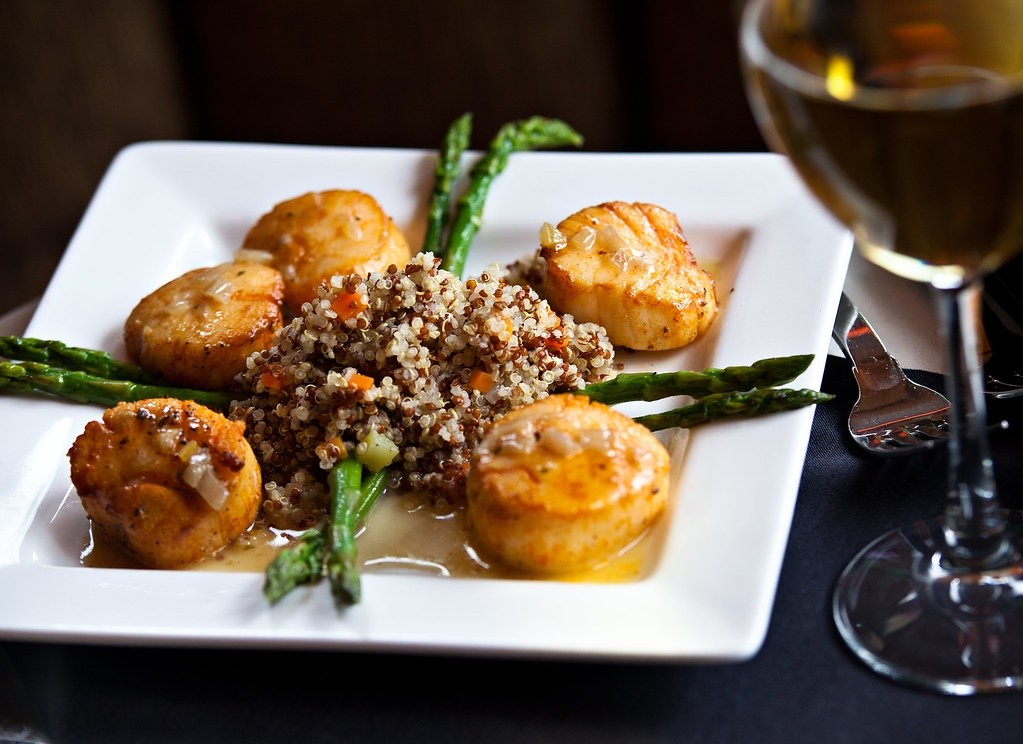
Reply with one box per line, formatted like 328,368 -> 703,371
0,142 -> 855,659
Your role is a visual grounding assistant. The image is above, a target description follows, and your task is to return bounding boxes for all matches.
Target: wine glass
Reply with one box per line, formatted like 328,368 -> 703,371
740,0 -> 1023,695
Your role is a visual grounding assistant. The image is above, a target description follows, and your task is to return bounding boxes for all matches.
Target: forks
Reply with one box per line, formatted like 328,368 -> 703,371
832,292 -> 1008,453
